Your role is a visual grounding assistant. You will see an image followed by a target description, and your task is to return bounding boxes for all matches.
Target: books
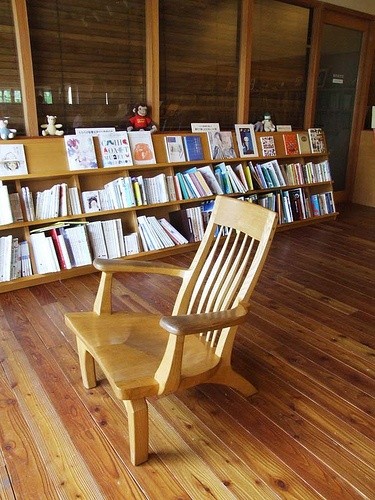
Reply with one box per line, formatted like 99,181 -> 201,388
0,161 -> 337,275
164,135 -> 186,163
0,144 -> 29,176
127,131 -> 158,165
297,132 -> 311,154
260,136 -> 275,157
207,130 -> 236,160
308,128 -> 325,154
98,130 -> 134,167
63,134 -> 98,170
235,123 -> 258,158
184,136 -> 204,161
283,134 -> 298,155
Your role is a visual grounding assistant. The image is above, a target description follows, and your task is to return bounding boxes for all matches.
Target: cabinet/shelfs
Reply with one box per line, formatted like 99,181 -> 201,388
0,128 -> 339,294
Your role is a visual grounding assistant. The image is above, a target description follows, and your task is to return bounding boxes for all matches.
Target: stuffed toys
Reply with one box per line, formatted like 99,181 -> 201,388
262,114 -> 276,132
254,121 -> 264,132
41,115 -> 64,137
127,103 -> 158,133
0,119 -> 17,140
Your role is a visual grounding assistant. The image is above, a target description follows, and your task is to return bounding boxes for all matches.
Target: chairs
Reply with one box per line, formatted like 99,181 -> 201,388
63,194 -> 280,467
